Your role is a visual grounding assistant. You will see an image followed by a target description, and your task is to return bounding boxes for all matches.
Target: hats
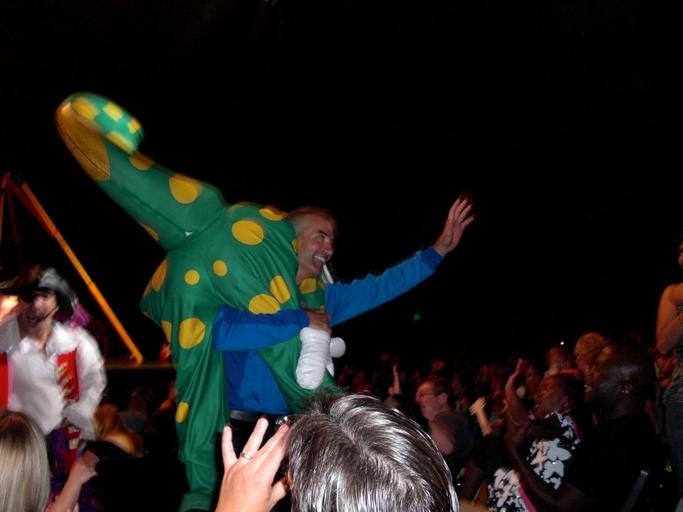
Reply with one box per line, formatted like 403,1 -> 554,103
1,266 -> 75,317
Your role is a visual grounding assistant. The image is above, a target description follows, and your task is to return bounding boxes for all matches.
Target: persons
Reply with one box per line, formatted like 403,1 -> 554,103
205,192 -> 482,512
1,267 -> 187,510
216,394 -> 459,512
335,283 -> 683,510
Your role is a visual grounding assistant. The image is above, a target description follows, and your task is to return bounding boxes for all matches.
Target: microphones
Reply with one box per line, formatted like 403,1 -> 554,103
35,306 -> 57,325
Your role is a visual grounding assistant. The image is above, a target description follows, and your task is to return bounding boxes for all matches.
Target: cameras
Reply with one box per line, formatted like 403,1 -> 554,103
210,409 -> 291,511
